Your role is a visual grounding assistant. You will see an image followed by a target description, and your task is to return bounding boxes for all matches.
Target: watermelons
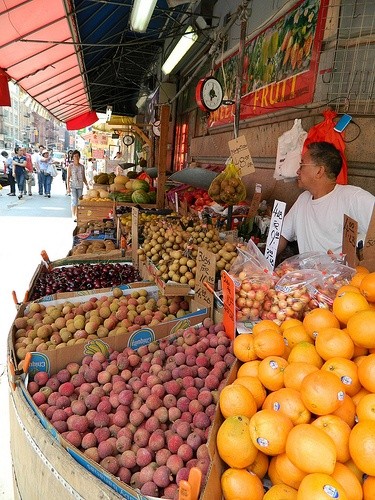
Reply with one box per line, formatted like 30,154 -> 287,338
109,179 -> 157,204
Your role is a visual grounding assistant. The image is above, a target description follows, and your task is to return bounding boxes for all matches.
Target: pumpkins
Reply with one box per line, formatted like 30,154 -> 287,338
109,174 -> 133,190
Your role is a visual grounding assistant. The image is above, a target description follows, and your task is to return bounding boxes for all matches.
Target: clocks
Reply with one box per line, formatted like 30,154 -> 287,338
122,135 -> 133,146
196,77 -> 224,111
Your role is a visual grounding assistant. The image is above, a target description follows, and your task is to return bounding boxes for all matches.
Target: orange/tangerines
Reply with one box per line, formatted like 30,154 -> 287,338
217,267 -> 375,500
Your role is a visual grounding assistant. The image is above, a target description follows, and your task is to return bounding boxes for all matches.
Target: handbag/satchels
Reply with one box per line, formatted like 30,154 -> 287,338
24,173 -> 29,180
32,176 -> 35,186
50,164 -> 58,177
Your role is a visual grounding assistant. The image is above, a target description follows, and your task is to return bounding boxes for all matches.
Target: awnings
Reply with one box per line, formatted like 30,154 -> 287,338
0,0 -> 188,130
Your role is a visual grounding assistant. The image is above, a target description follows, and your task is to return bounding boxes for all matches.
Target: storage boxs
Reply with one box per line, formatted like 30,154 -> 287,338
3,200 -> 294,500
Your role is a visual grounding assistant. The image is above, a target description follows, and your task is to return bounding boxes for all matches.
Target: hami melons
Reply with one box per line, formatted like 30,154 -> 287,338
116,204 -> 156,214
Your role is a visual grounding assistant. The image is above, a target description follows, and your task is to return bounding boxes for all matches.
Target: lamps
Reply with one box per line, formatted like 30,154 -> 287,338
127,0 -> 200,76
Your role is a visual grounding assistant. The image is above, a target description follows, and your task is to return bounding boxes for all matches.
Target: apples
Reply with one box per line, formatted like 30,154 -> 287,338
120,213 -> 177,227
182,187 -> 248,212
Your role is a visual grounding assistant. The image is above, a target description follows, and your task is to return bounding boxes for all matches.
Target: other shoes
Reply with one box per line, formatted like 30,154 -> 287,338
7,193 -> 15,196
18,195 -> 22,199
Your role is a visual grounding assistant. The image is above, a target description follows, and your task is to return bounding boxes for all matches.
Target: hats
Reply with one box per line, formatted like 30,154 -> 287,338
42,150 -> 48,156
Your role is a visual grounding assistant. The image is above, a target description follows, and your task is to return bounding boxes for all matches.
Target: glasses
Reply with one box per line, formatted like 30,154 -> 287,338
299,162 -> 315,169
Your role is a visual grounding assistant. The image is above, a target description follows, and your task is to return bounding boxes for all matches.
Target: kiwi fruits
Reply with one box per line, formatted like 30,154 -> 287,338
76,221 -> 113,234
73,240 -> 115,256
209,177 -> 244,203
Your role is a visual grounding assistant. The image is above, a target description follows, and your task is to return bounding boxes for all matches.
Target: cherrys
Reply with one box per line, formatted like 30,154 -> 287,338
230,261 -> 345,326
31,258 -> 142,302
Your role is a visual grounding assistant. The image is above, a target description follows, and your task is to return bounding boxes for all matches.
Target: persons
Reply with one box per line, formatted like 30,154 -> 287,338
40,149 -> 55,198
63,150 -> 74,196
67,150 -> 89,222
260,141 -> 375,269
22,147 -> 33,196
1,151 -> 16,196
113,152 -> 125,160
88,158 -> 97,185
12,146 -> 27,200
34,145 -> 46,195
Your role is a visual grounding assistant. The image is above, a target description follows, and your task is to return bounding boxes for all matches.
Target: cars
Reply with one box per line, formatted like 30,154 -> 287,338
0,150 -> 17,183
53,152 -> 66,169
